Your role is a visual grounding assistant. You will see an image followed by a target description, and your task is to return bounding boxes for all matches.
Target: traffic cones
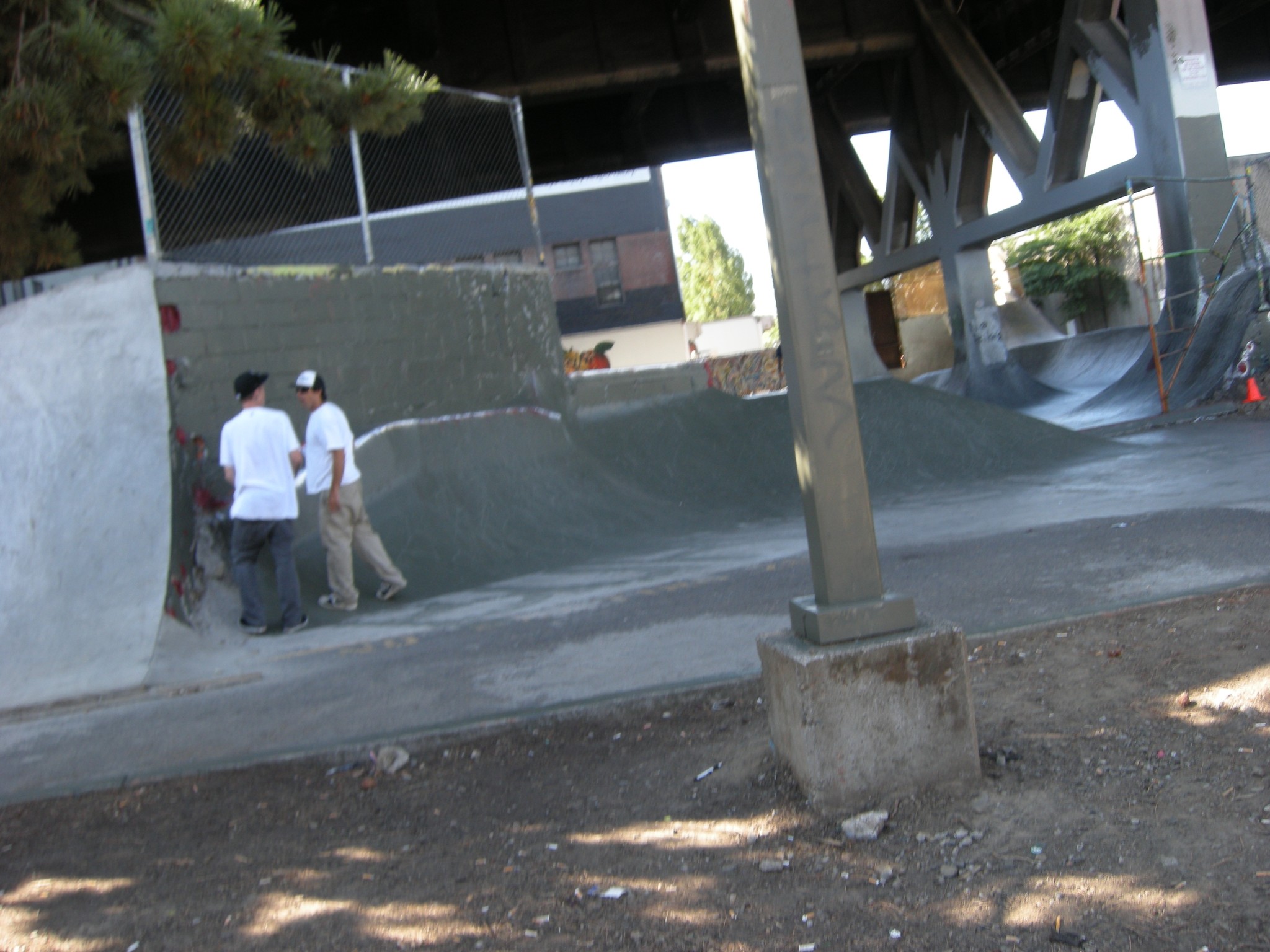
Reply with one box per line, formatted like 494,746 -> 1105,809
1243,378 -> 1267,404
687,340 -> 700,362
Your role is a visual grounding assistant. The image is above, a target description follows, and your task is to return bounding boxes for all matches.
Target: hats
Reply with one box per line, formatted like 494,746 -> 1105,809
294,370 -> 325,392
234,371 -> 268,400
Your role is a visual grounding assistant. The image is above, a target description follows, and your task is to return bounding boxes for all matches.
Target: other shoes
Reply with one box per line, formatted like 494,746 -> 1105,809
239,616 -> 267,634
318,595 -> 357,611
376,578 -> 407,601
283,614 -> 309,633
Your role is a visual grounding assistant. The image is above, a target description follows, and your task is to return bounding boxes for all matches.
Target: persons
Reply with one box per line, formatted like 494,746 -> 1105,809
217,370 -> 311,634
775,345 -> 783,372
289,368 -> 408,611
